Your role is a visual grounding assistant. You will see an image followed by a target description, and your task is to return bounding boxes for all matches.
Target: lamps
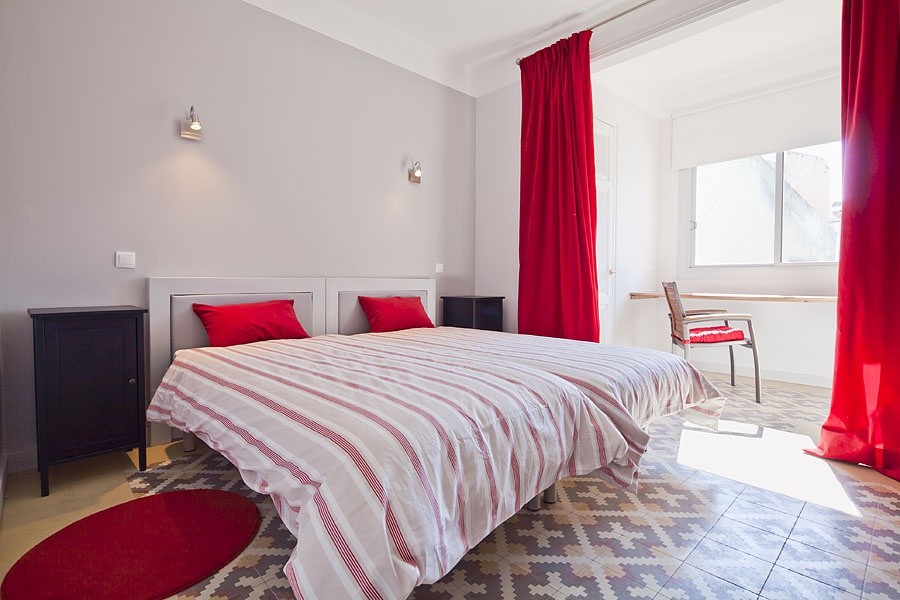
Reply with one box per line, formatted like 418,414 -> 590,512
408,161 -> 421,182
180,105 -> 202,140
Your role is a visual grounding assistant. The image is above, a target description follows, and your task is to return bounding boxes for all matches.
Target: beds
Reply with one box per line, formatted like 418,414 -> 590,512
147,275 -> 729,599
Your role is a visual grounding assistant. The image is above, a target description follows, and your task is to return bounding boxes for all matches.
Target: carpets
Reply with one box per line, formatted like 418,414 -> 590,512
0,489 -> 259,600
125,376 -> 900,600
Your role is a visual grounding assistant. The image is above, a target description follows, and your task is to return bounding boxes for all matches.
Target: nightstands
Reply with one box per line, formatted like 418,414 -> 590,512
441,295 -> 505,332
27,304 -> 149,498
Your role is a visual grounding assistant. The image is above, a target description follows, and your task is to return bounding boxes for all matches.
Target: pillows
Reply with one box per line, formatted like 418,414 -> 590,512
358,295 -> 435,332
192,299 -> 311,346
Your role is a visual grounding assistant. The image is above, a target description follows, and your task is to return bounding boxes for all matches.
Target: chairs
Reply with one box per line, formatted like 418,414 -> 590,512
661,279 -> 761,403
